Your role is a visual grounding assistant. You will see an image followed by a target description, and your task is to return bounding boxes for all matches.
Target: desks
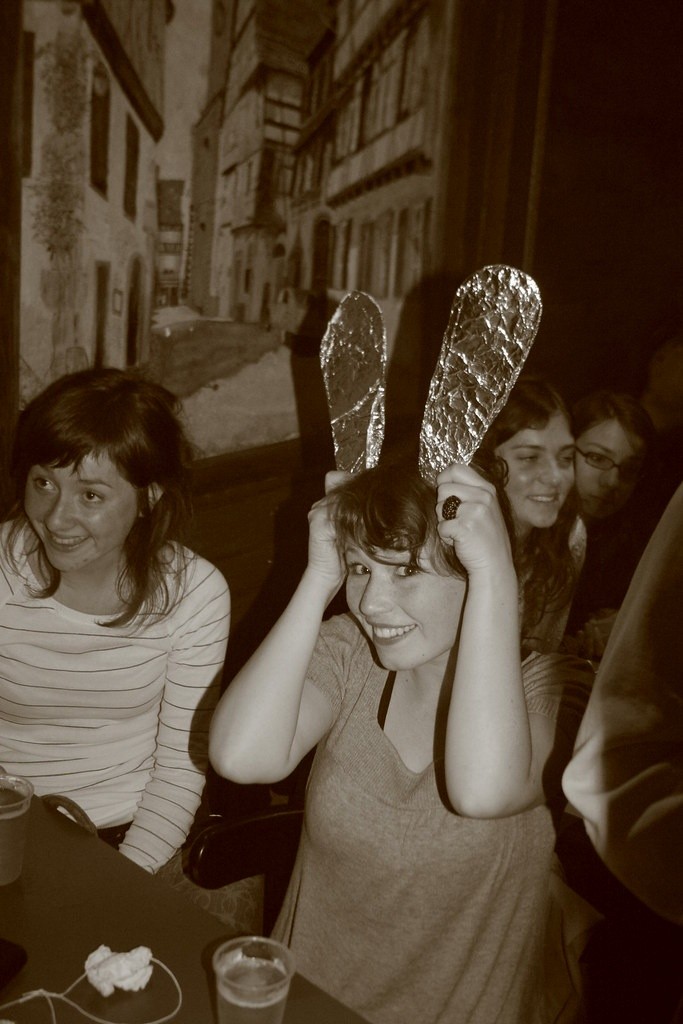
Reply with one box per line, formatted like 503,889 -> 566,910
0,786 -> 367,1024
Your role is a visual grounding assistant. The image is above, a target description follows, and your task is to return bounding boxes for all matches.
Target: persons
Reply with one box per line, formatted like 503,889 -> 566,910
472,378 -> 576,658
562,486 -> 683,1020
0,372 -> 238,876
552,392 -> 658,770
208,462 -> 574,1024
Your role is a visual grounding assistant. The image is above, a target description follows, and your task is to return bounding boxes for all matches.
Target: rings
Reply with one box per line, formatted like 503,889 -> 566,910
442,495 -> 461,519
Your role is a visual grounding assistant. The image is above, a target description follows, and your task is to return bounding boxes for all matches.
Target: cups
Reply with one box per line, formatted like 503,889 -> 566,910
213,937 -> 295,1024
0,774 -> 34,886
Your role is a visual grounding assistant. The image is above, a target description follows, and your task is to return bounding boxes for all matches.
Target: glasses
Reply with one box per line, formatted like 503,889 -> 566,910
573,444 -> 646,486
